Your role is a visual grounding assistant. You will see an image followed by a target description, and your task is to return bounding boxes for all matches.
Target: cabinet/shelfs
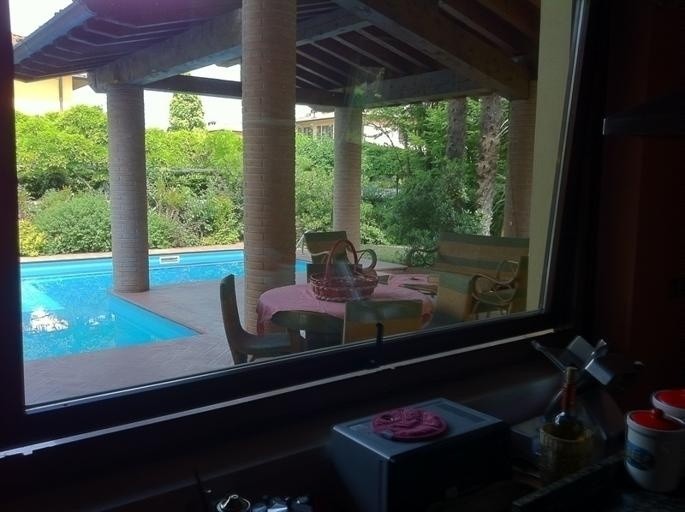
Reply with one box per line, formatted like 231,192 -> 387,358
594,0 -> 685,415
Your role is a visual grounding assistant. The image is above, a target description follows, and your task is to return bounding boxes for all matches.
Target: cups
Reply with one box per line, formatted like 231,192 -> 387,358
538,425 -> 599,471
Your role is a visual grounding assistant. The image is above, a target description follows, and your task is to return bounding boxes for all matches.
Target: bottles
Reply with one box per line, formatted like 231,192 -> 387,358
552,366 -> 585,438
652,388 -> 684,420
623,407 -> 684,495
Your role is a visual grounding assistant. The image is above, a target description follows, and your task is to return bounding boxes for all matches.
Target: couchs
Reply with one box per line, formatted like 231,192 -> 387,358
405,231 -> 529,320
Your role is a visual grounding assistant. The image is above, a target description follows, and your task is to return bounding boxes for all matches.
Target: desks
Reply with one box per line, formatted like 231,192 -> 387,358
259,283 -> 430,352
329,432 -> 685,512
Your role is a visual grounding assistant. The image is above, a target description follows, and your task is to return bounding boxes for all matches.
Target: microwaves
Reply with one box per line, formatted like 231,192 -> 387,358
327,398 -> 511,512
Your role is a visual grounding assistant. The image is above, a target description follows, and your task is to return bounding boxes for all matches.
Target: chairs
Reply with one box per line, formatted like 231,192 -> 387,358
469,255 -> 528,321
220,274 -> 304,366
343,300 -> 423,345
304,231 -> 377,273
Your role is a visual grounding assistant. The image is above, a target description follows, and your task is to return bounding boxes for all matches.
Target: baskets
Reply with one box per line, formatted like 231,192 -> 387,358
311,240 -> 378,302
